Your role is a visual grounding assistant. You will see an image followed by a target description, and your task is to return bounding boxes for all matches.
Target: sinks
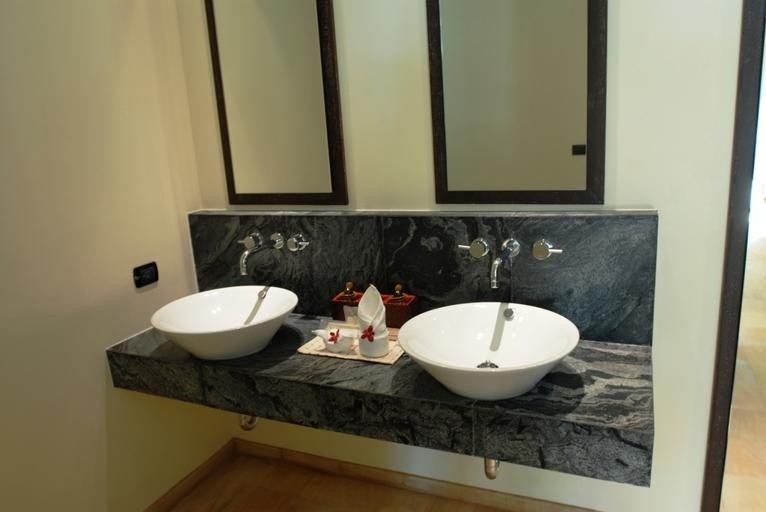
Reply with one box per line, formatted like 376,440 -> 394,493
398,300 -> 580,402
150,285 -> 299,361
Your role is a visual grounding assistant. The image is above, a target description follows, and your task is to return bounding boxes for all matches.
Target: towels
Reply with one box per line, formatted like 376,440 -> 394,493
309,282 -> 390,358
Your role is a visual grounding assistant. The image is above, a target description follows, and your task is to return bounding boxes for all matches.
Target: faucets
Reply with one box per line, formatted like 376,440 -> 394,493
488,238 -> 521,290
238,231 -> 285,279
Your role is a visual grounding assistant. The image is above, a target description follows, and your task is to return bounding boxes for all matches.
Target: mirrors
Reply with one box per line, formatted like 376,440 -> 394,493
201,1 -> 349,206
424,0 -> 608,206
